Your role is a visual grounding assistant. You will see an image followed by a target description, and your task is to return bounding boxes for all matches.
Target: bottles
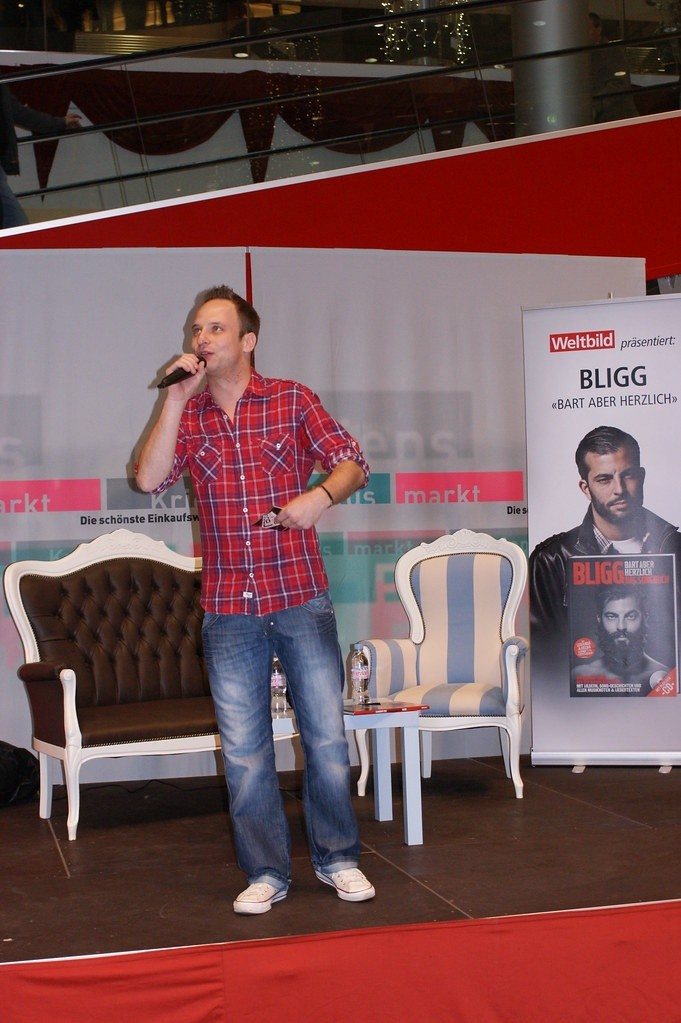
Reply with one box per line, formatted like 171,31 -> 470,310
350,644 -> 370,705
271,658 -> 286,714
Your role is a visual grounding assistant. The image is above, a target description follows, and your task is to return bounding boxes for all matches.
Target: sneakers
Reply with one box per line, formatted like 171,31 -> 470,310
233,882 -> 288,916
315,867 -> 375,901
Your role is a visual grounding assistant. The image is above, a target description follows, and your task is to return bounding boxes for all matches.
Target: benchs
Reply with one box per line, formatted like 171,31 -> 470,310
4,529 -> 222,840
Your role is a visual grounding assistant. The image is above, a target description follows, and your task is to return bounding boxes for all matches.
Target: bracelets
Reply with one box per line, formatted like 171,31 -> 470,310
319,484 -> 335,508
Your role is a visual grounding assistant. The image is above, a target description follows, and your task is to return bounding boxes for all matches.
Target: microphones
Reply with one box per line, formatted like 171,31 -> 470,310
157,356 -> 207,389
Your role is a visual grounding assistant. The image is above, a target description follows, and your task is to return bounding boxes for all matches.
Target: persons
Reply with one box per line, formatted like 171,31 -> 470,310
528,426 -> 680,668
571,582 -> 669,697
136,285 -> 376,913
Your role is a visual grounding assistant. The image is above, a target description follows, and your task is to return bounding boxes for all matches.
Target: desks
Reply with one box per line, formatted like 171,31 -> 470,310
271,697 -> 423,845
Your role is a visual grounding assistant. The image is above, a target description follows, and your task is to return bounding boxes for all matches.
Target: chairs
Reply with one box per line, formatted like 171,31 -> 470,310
356,529 -> 526,799
